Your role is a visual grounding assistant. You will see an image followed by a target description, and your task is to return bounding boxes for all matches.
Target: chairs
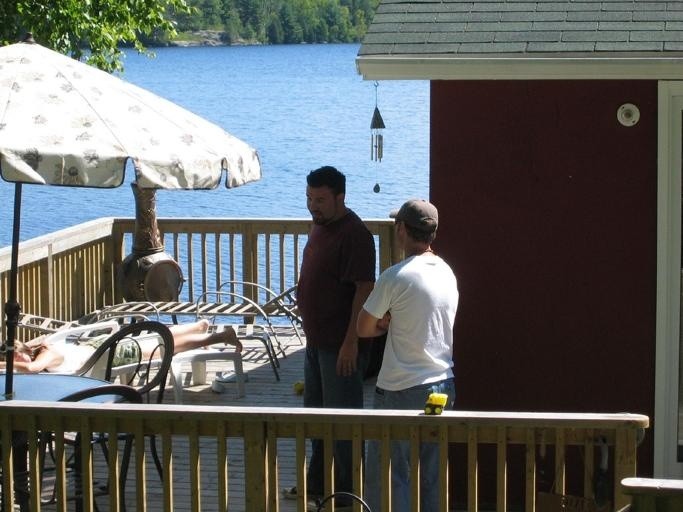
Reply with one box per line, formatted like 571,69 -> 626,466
4,311 -> 282,382
21,386 -> 143,512
88,281 -> 302,359
48,321 -> 175,482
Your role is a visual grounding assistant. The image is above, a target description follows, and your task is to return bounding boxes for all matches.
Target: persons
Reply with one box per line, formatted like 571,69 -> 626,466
356,198 -> 462,494
284,166 -> 376,494
1,316 -> 242,373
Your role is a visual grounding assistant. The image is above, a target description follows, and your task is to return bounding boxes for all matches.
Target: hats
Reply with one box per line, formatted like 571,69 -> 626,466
389,199 -> 440,232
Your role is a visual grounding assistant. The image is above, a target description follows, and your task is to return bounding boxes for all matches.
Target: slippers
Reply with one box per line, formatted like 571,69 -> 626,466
215,370 -> 248,383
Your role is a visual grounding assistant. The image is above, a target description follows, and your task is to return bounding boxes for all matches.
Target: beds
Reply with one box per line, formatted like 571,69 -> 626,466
0,319 -> 246,406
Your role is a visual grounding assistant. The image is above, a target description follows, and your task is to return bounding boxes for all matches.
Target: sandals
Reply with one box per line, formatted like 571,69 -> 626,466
211,380 -> 225,393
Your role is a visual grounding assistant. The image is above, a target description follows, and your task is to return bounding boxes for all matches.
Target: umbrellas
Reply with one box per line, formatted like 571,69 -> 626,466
0,32 -> 263,399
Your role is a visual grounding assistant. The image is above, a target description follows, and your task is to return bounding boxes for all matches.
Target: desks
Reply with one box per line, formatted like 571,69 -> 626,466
0,373 -> 125,512
